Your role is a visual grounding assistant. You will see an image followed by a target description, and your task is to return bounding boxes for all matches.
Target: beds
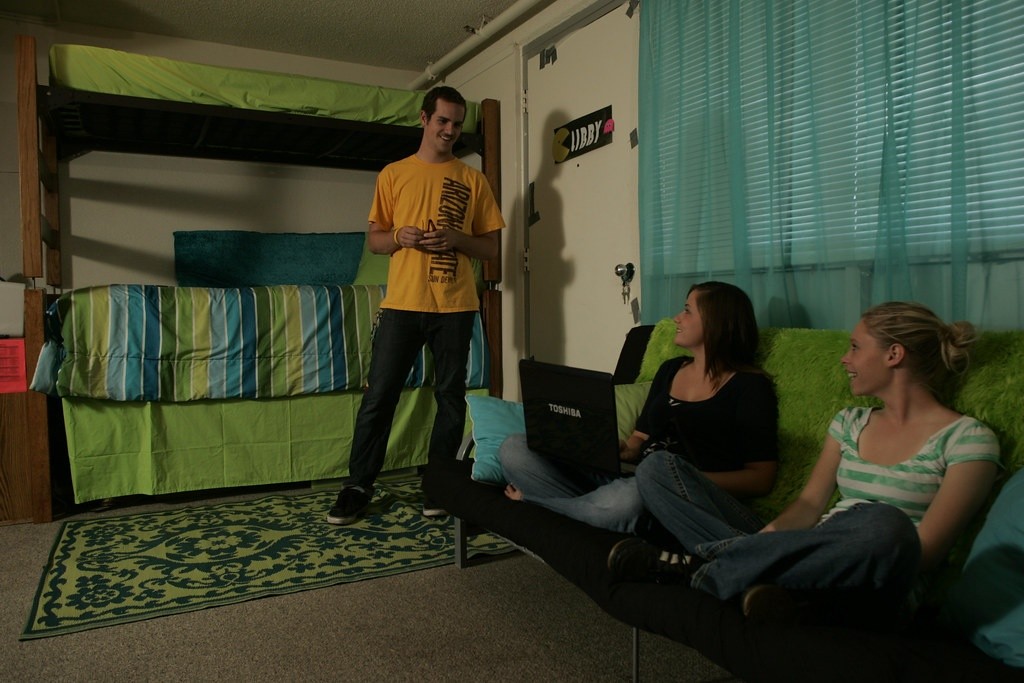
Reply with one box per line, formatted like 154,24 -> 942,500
16,287 -> 503,513
16,30 -> 502,282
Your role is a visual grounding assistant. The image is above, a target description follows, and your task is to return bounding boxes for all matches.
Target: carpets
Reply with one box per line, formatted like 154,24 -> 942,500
18,472 -> 518,641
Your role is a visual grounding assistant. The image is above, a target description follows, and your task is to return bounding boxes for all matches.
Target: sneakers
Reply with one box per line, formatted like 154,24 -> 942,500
326,483 -> 369,524
744,587 -> 779,616
606,538 -> 700,582
422,487 -> 446,516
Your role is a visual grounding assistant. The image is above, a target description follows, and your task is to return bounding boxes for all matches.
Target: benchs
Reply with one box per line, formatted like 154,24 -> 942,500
426,321 -> 1024,683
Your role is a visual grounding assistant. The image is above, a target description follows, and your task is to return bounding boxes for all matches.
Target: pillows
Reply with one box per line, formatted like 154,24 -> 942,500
464,394 -> 524,483
357,230 -> 390,284
940,468 -> 1024,667
173,225 -> 363,286
615,381 -> 652,446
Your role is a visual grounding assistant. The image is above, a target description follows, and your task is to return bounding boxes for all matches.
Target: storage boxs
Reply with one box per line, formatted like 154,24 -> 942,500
0,336 -> 27,393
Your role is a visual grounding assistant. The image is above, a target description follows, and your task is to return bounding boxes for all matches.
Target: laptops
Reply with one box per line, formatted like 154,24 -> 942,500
518,359 -> 640,478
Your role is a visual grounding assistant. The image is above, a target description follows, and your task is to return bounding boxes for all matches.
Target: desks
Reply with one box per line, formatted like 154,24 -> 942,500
0,393 -> 53,524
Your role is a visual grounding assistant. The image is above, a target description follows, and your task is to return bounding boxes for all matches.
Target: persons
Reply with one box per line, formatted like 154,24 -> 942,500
500,281 -> 778,530
607,301 -> 1007,627
326,86 -> 506,524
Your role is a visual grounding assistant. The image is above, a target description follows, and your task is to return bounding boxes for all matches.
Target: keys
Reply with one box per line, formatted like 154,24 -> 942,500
621,292 -> 627,304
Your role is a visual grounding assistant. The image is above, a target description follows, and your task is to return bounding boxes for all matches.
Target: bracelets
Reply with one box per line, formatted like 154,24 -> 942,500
394,226 -> 402,246
